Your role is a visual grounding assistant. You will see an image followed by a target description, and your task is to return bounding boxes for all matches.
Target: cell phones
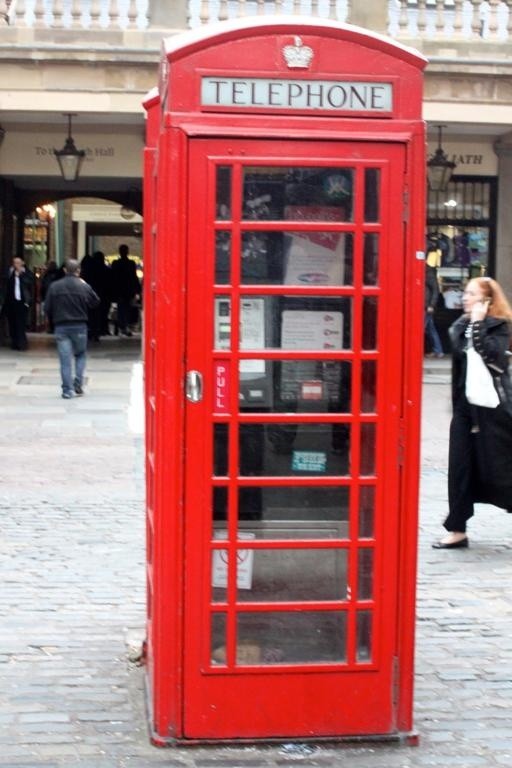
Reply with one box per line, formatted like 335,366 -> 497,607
480,296 -> 492,311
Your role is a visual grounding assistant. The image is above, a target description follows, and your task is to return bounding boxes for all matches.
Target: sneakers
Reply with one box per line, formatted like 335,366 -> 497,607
426,352 -> 445,358
73,386 -> 84,395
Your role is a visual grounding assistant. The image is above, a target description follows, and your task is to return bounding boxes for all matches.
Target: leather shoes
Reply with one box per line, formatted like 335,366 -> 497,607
433,537 -> 468,549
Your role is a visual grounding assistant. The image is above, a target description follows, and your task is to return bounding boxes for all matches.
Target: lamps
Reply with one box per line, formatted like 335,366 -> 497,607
51,112 -> 85,183
427,125 -> 457,193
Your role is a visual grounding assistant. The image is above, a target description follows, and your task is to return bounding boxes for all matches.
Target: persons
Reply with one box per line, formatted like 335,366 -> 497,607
431,275 -> 512,550
0,242 -> 145,350
423,259 -> 444,360
264,168 -> 374,456
45,257 -> 102,398
